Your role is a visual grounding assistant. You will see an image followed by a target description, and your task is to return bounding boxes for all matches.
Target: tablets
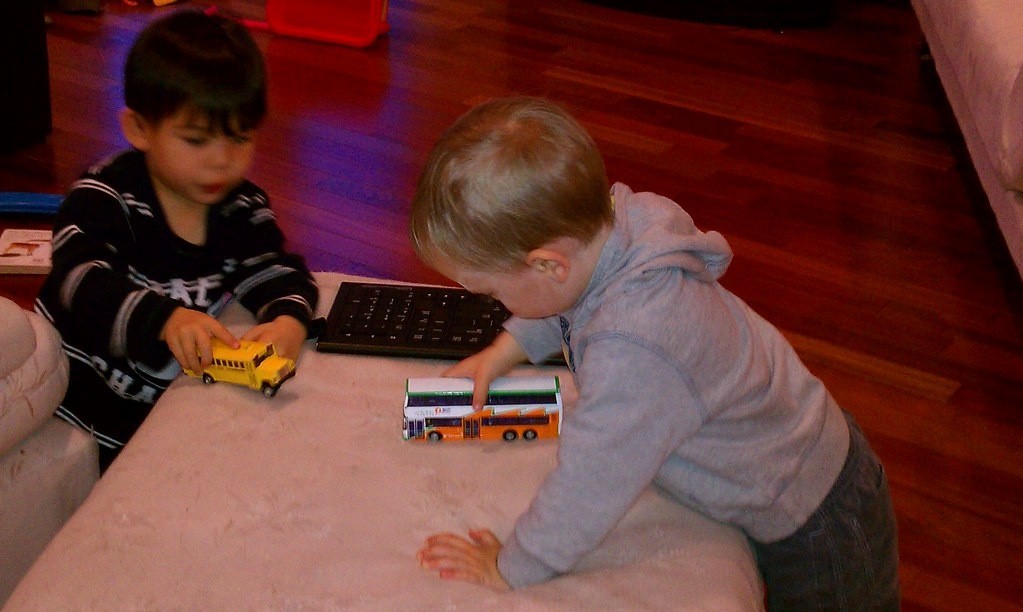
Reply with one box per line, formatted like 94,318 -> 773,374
316,282 -> 569,368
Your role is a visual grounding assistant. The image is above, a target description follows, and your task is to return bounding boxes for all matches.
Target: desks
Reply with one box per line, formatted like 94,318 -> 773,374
3,271 -> 768,612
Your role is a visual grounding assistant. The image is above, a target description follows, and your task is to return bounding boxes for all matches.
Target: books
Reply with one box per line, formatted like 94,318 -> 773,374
0,228 -> 53,274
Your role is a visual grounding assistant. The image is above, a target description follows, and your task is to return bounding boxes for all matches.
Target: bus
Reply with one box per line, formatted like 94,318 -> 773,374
181,338 -> 299,398
400,374 -> 563,441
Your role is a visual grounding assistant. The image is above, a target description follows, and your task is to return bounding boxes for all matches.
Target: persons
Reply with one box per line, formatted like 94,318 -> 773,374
34,10 -> 320,471
406,99 -> 901,612
